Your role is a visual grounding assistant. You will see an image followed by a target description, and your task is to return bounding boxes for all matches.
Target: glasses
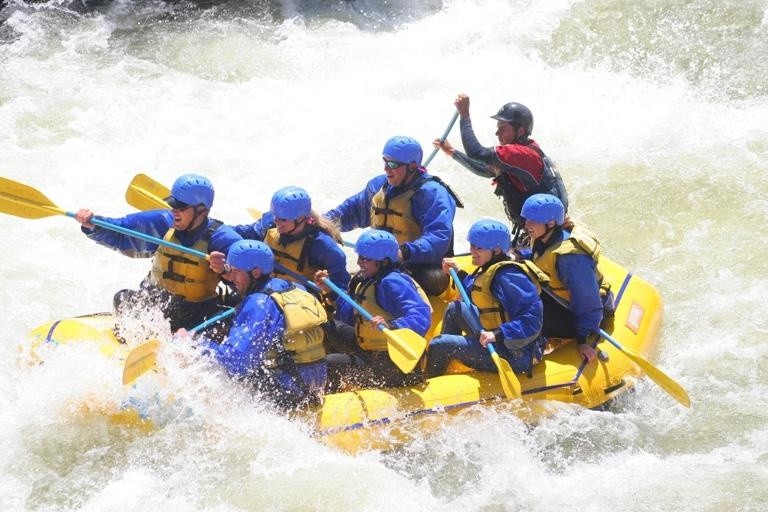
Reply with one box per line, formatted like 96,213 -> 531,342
180,206 -> 193,212
359,255 -> 372,263
383,157 -> 409,170
281,218 -> 287,222
471,244 -> 482,250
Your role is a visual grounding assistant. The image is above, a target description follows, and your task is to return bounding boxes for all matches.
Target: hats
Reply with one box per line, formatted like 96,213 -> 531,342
163,174 -> 214,209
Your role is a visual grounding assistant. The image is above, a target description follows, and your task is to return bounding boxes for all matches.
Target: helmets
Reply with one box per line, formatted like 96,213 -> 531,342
382,136 -> 423,167
226,240 -> 274,274
489,102 -> 533,135
270,186 -> 311,221
520,193 -> 565,226
466,219 -> 510,254
355,230 -> 399,264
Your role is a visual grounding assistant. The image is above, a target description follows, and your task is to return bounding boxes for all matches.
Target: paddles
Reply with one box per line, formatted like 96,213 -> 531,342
121,306 -> 236,386
448,267 -> 522,403
541,285 -> 691,407
319,274 -> 427,376
124,174 -> 174,210
249,207 -> 355,251
0,176 -> 210,264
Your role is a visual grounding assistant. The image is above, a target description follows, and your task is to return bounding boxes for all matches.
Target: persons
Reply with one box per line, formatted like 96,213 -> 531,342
177,238 -> 328,411
425,218 -> 545,376
312,231 -> 434,392
319,136 -> 458,297
432,91 -> 574,243
514,192 -> 613,363
211,186 -> 352,306
73,173 -> 244,344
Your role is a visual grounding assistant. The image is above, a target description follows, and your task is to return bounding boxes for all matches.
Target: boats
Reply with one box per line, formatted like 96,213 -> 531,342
31,246 -> 660,455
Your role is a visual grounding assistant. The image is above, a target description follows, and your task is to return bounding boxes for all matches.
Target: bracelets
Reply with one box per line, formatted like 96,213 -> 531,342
218,269 -> 228,277
